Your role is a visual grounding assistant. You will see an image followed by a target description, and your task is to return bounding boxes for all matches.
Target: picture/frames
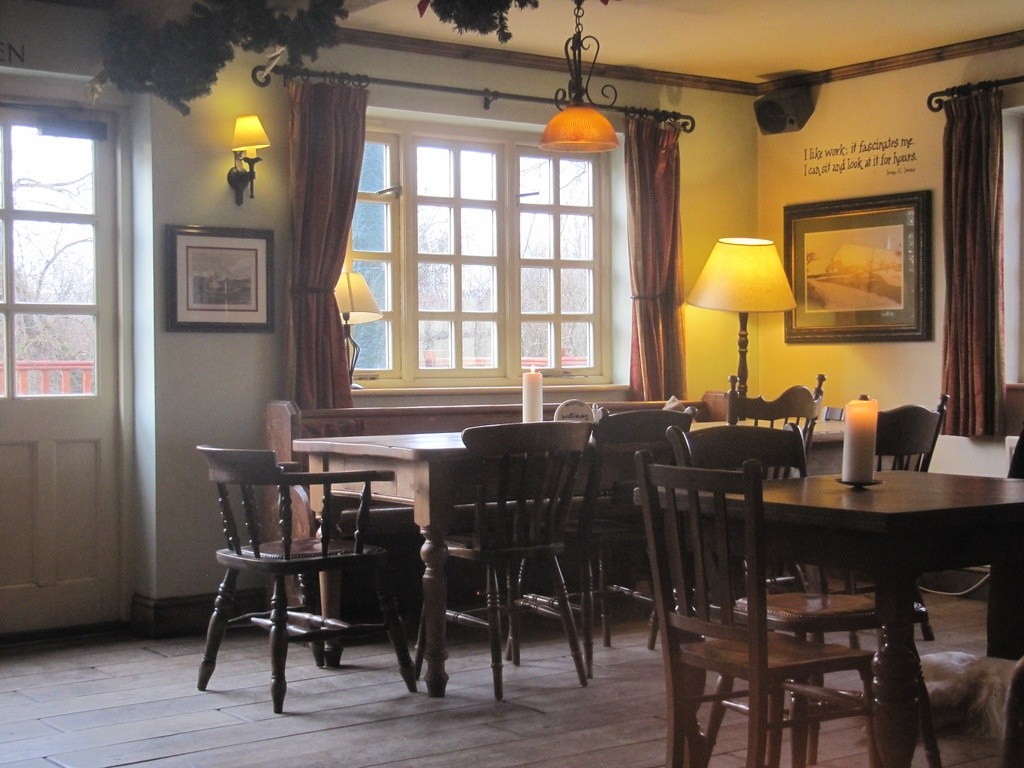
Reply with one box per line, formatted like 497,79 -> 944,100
165,223 -> 275,333
782,190 -> 932,343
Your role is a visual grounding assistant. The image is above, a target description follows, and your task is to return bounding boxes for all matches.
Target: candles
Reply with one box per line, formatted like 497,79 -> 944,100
841,398 -> 879,484
522,371 -> 544,423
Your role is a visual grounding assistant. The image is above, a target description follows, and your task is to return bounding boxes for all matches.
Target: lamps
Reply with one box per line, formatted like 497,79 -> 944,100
228,115 -> 271,205
333,272 -> 383,389
538,0 -> 619,153
685,237 -> 796,420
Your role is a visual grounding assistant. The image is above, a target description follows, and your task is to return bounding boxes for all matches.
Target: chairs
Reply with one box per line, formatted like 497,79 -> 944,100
192,373 -> 1024,768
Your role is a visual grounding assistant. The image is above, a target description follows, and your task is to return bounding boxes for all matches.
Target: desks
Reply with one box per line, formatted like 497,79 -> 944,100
632,469 -> 1024,768
291,430 -> 840,696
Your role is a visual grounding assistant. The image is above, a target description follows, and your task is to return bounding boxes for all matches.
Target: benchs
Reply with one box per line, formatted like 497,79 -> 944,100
262,393 -> 729,631
